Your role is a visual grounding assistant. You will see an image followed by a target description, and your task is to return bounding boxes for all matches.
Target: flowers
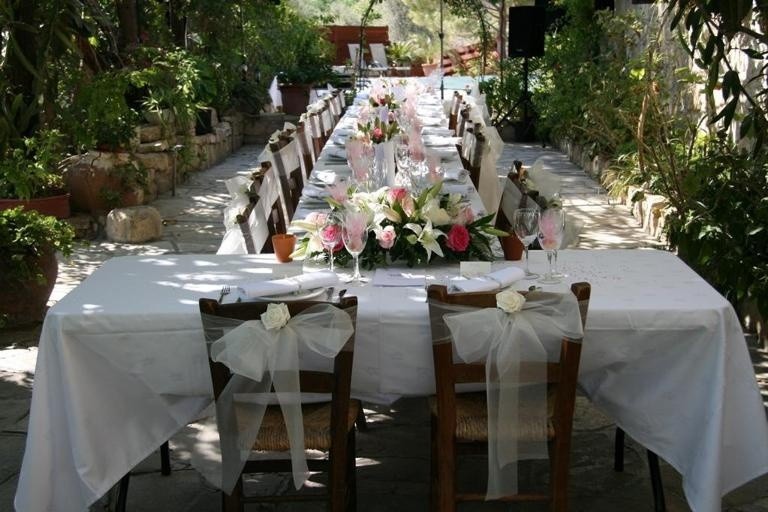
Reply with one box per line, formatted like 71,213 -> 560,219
332,89 -> 443,201
260,301 -> 290,328
494,288 -> 523,312
287,176 -> 514,276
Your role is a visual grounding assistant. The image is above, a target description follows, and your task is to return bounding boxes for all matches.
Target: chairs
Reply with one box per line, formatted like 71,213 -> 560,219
446,90 -> 545,254
236,89 -> 347,253
198,296 -> 364,510
419,281 -> 591,511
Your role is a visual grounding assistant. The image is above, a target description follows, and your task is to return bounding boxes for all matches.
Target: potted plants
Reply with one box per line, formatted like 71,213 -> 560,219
277,11 -> 337,114
2,125 -> 76,330
386,30 -> 438,77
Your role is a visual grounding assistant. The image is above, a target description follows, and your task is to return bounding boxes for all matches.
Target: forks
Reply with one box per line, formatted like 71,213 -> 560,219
218,285 -> 230,305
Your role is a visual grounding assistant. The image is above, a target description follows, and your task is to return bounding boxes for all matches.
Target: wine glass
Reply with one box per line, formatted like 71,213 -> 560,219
512,207 -> 565,285
315,210 -> 371,288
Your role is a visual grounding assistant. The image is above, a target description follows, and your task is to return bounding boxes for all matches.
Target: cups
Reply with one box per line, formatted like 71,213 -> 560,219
271,234 -> 297,262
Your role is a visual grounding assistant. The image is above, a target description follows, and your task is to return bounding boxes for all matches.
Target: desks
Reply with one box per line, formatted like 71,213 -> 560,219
45,247 -> 741,511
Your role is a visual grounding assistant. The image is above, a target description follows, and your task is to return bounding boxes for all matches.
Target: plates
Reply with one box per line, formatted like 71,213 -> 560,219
287,92 -> 482,235
254,287 -> 326,302
